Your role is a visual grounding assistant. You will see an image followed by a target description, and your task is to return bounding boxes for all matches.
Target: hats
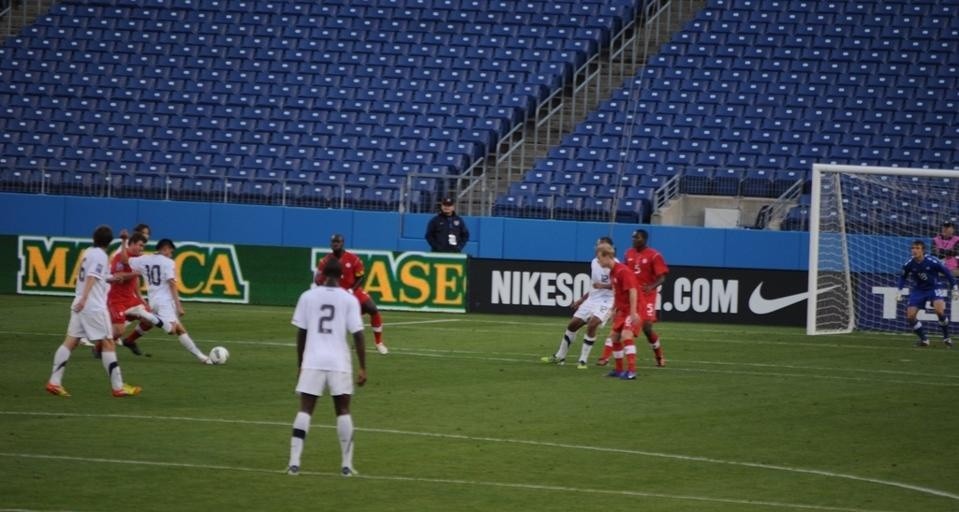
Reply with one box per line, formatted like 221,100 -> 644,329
941,221 -> 952,226
443,197 -> 453,205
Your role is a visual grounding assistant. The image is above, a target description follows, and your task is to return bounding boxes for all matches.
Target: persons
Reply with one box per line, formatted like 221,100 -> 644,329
92,232 -> 156,358
424,195 -> 469,253
118,227 -> 213,366
45,225 -> 143,397
930,219 -> 958,289
79,222 -> 151,349
895,239 -> 956,349
594,243 -> 648,380
598,228 -> 669,369
284,256 -> 368,478
315,232 -> 389,357
539,236 -> 620,372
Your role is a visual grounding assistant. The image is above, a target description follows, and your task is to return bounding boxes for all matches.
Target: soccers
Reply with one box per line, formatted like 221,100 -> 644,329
210,347 -> 230,365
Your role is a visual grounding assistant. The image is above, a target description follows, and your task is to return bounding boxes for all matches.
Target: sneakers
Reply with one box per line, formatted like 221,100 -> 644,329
282,465 -> 300,475
656,356 -> 663,366
913,340 -> 929,348
596,359 -> 607,366
123,338 -> 141,355
577,361 -> 587,369
112,383 -> 142,396
45,383 -> 71,397
80,338 -> 95,346
375,343 -> 388,355
541,356 -> 564,365
339,466 -> 356,476
944,338 -> 952,346
116,338 -> 122,345
606,369 -> 623,377
625,370 -> 636,380
